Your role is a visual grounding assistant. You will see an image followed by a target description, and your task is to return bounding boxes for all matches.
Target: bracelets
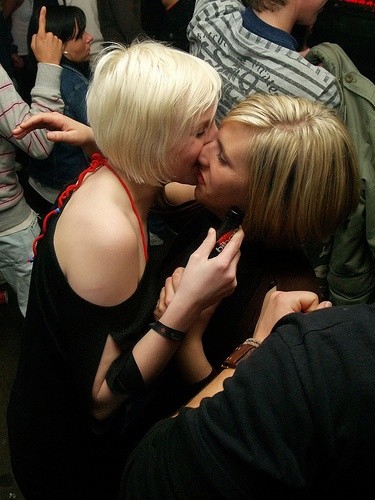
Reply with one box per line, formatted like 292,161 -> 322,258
220,338 -> 261,369
151,320 -> 188,344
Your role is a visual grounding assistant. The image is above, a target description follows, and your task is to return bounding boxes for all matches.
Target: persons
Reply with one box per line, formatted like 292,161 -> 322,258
19,5 -> 93,230
11,92 -> 364,421
185,0 -> 342,129
0,0 -> 107,75
0,6 -> 63,321
119,290 -> 375,500
7,43 -> 247,500
15,5 -> 95,224
308,0 -> 375,85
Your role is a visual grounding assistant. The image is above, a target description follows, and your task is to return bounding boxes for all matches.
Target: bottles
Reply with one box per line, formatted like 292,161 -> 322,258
208,205 -> 245,260
